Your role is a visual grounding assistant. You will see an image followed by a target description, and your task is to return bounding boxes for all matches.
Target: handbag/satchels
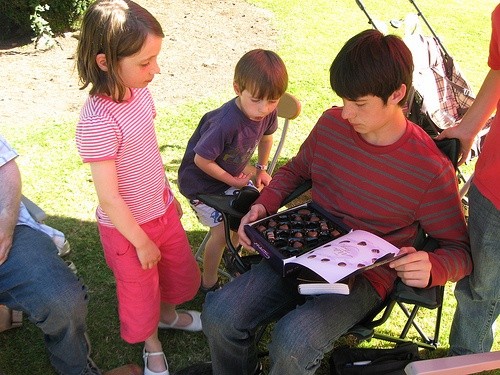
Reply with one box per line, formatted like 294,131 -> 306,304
330,341 -> 420,375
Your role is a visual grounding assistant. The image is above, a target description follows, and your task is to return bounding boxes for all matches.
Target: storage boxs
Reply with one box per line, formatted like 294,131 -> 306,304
244,200 -> 401,284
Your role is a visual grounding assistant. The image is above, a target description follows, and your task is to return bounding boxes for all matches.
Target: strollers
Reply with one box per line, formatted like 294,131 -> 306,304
356,0 -> 493,208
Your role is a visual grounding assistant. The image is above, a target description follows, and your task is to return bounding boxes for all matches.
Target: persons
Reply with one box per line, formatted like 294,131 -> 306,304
0,134 -> 144,375
434,1 -> 500,358
75,0 -> 203,375
178,49 -> 289,297
201,29 -> 474,375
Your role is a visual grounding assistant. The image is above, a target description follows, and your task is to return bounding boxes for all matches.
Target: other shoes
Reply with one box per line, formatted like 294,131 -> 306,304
142,343 -> 170,375
157,308 -> 203,331
199,277 -> 222,294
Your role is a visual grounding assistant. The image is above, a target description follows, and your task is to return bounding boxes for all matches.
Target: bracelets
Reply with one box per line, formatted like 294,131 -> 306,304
256,163 -> 268,170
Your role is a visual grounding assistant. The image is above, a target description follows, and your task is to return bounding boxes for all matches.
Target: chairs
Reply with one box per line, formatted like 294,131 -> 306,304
195,138 -> 461,359
195,91 -> 302,283
